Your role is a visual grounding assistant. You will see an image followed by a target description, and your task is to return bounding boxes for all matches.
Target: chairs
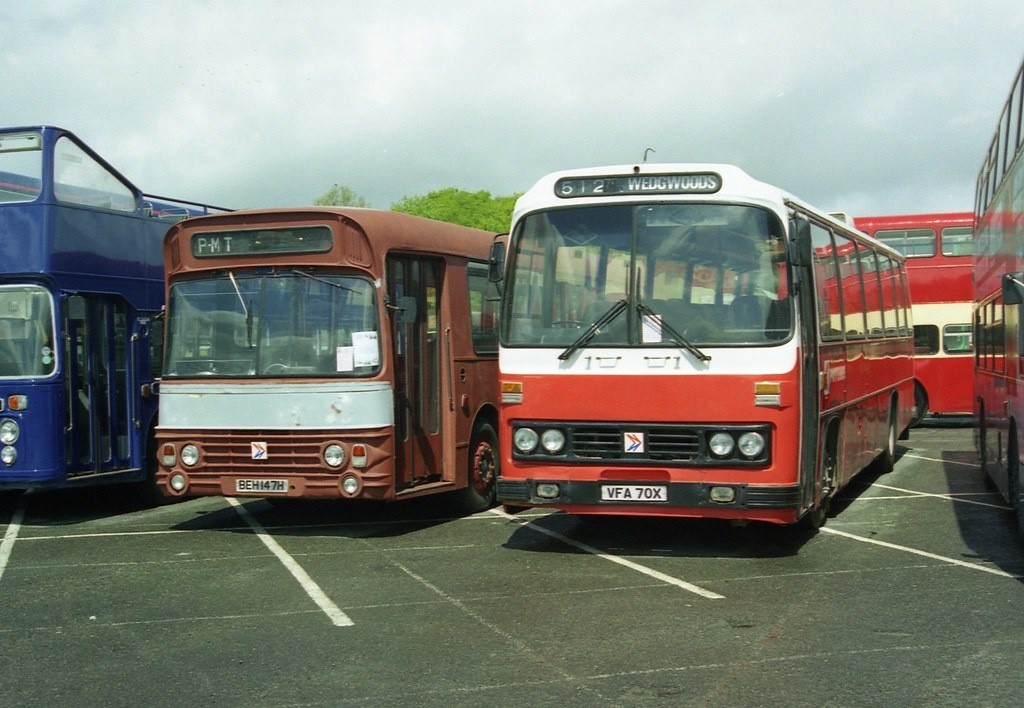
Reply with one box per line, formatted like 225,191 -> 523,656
584,294 -> 792,341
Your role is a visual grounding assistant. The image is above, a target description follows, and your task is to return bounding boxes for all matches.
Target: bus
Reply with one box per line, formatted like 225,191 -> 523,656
780,210 -> 976,426
489,162 -> 916,541
0,125 -> 403,493
149,207 -> 547,511
972,62 -> 1024,533
